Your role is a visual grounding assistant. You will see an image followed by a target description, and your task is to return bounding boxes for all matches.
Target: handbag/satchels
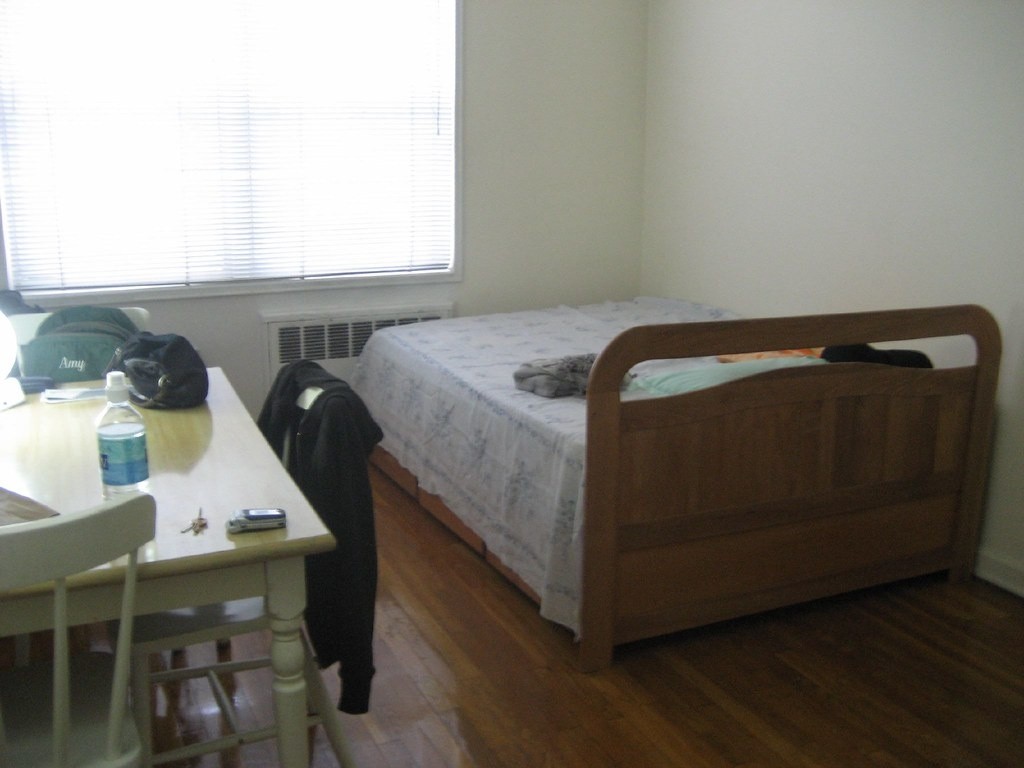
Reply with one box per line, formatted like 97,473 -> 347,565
101,331 -> 209,409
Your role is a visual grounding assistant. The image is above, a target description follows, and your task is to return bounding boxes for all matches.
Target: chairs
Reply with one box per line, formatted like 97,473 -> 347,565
0,490 -> 156,768
109,359 -> 384,768
8,306 -> 152,378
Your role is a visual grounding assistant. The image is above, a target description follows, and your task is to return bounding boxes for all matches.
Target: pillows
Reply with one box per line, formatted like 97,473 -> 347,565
633,357 -> 832,397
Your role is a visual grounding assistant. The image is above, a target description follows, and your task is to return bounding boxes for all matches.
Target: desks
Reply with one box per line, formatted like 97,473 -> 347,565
0,365 -> 339,768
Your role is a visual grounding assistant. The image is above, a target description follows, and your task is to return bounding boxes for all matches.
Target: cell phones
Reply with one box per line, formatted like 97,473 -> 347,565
226,508 -> 286,534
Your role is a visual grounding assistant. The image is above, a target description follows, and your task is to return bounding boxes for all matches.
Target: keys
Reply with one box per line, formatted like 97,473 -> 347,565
181,506 -> 208,535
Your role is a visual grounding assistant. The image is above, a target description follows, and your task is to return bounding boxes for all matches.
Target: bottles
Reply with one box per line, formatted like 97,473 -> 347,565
96,371 -> 149,505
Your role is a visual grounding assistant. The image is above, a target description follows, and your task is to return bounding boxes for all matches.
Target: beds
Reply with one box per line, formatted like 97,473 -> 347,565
346,293 -> 1004,676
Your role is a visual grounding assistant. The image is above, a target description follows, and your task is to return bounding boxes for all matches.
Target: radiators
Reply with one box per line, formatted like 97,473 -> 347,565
257,301 -> 454,398
